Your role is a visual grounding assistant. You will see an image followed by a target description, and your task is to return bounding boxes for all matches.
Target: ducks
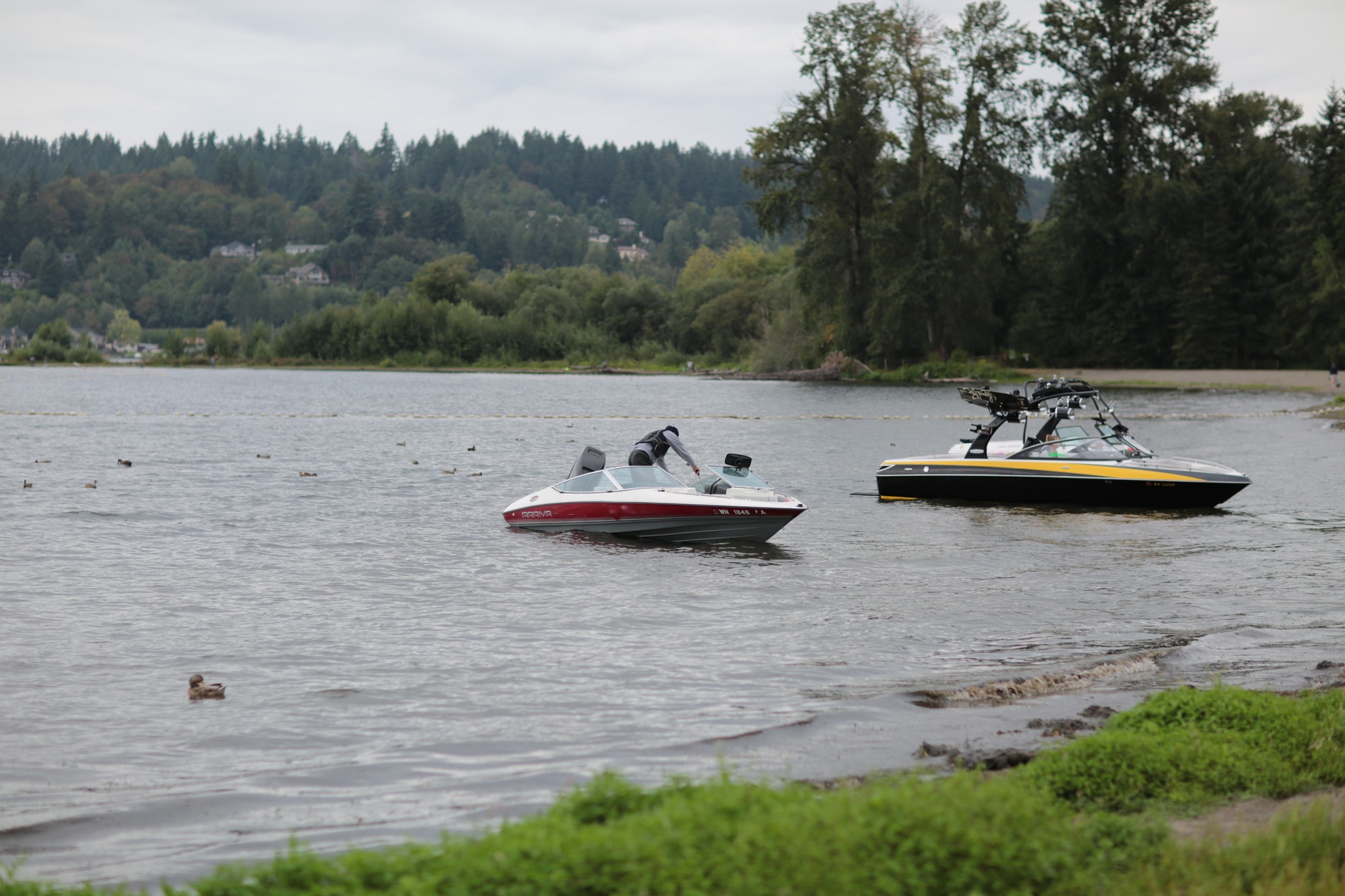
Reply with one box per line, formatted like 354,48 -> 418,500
188,674 -> 228,698
23,423 -> 577,488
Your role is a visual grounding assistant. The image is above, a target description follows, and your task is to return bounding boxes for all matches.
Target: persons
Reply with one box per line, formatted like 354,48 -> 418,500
1329,363 -> 1338,387
628,426 -> 700,483
1038,435 -> 1061,458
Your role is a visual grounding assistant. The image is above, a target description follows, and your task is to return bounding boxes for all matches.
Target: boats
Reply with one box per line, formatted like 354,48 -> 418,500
872,374 -> 1255,514
500,446 -> 810,545
